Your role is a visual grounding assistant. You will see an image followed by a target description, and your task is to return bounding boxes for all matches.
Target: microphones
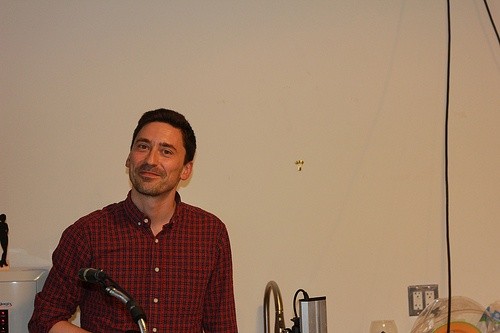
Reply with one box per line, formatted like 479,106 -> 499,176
78,268 -> 106,282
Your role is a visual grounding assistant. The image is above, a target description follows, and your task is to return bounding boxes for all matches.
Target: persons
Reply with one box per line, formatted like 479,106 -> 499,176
0,214 -> 9,268
27,108 -> 239,333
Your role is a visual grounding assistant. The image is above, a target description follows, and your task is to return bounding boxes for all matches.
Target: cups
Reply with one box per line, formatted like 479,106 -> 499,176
370,320 -> 398,333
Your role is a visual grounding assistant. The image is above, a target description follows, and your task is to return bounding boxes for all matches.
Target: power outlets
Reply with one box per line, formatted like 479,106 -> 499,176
408,284 -> 438,316
